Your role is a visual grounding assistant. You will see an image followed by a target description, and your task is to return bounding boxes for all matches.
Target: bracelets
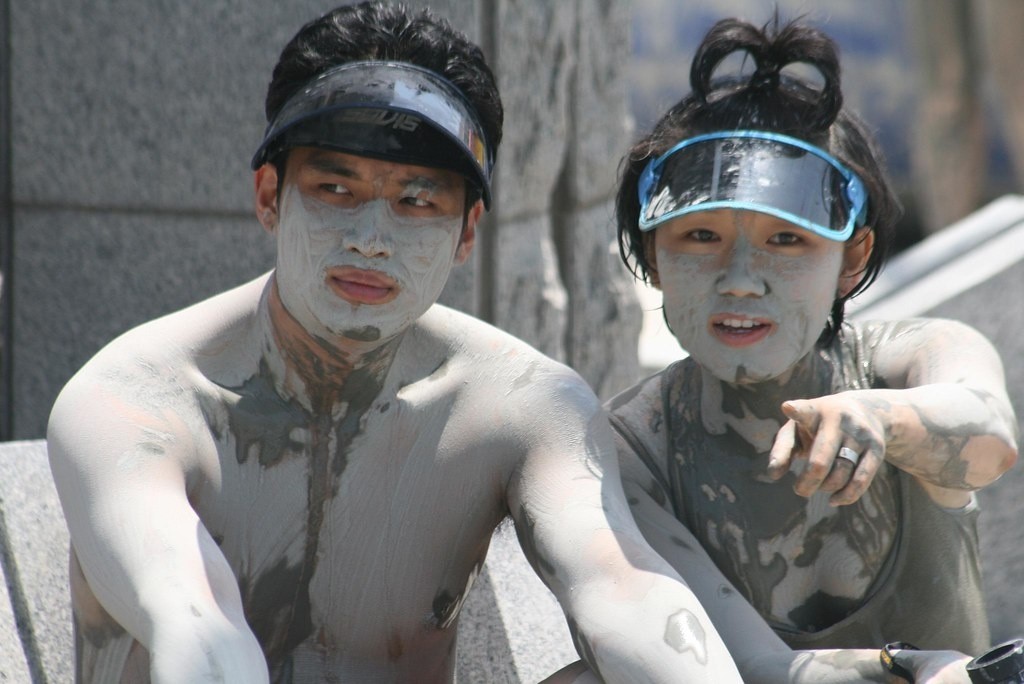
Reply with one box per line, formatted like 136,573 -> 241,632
880,639 -> 920,684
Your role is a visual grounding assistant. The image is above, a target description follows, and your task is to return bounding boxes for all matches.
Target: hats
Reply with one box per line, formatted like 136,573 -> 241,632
252,62 -> 493,211
635,131 -> 870,241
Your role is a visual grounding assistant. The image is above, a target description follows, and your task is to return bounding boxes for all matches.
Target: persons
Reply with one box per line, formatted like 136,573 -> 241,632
46,0 -> 747,684
595,1 -> 1019,684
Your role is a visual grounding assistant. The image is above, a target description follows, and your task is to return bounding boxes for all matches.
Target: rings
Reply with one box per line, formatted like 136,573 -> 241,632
838,446 -> 859,466
